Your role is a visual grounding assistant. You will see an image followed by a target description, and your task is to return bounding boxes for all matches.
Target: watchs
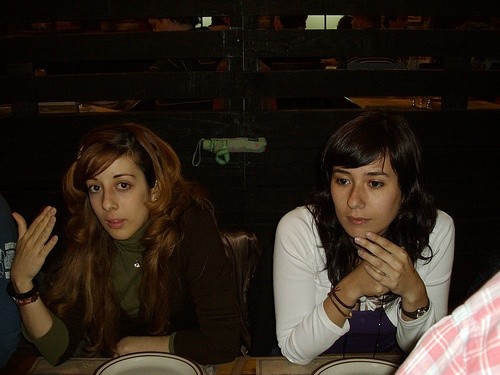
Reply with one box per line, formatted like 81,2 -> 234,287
399,299 -> 430,319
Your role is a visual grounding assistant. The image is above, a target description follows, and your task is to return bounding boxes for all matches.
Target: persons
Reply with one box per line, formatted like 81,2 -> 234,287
272,111 -> 455,365
0,120 -> 242,375
0,0 -> 500,70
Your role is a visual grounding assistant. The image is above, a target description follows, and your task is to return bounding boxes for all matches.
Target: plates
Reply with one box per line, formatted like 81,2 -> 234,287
94,351 -> 203,375
311,357 -> 400,375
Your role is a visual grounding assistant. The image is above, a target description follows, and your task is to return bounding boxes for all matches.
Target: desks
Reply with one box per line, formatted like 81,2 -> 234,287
344,95 -> 500,110
0,101 -> 122,117
0,352 -> 407,375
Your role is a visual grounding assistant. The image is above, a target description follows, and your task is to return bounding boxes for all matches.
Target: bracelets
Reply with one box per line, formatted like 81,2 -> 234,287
328,286 -> 356,319
7,283 -> 40,306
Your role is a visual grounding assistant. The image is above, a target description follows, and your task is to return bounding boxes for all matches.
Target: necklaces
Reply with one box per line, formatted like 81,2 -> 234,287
117,242 -> 145,268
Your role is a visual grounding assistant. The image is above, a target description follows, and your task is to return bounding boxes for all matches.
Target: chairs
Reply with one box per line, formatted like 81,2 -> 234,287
221,230 -> 261,357
271,57 -> 398,109
